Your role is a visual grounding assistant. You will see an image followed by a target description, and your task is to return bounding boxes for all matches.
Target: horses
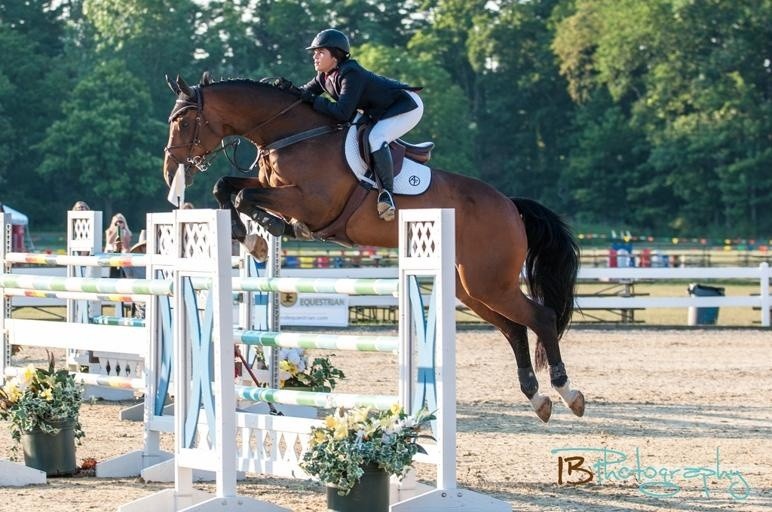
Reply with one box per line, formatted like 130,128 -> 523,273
160,70 -> 586,424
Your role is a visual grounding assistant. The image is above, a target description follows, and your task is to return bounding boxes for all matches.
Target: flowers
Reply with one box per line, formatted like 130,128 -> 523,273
295,397 -> 441,498
1,347 -> 86,462
277,346 -> 346,393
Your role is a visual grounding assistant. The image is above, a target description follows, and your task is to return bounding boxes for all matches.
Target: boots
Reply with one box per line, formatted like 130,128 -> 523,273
370,143 -> 396,221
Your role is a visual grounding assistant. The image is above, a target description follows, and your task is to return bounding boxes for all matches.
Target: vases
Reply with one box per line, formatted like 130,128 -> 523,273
21,418 -> 76,477
326,463 -> 391,512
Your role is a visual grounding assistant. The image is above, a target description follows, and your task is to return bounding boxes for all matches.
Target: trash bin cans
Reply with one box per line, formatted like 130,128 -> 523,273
689,283 -> 723,326
662,256 -> 669,268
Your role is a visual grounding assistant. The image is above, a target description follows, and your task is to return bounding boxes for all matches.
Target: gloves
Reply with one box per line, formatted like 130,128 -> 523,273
273,76 -> 316,107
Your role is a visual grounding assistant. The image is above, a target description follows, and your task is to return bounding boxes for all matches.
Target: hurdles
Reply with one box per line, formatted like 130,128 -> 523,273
175,206 -> 509,512
1,206 -> 287,484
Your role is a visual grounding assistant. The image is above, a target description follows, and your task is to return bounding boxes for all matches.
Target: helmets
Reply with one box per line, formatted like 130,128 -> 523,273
306,29 -> 351,55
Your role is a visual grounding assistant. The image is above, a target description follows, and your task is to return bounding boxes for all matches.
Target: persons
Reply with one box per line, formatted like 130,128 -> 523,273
130,228 -> 147,254
293,26 -> 424,224
71,199 -> 96,257
104,214 -> 134,277
